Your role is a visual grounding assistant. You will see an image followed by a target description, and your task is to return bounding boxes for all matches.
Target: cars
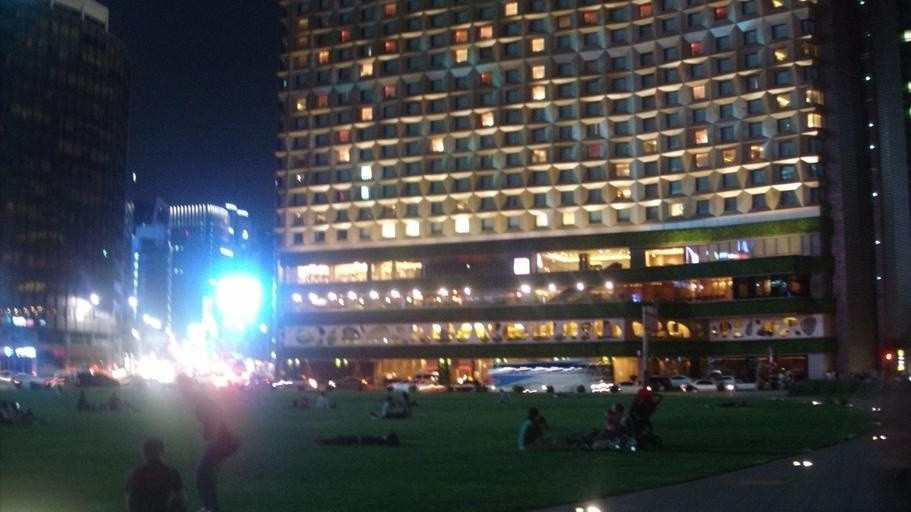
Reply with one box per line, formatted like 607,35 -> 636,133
272,370 -> 476,395
613,373 -> 758,395
0,369 -> 161,390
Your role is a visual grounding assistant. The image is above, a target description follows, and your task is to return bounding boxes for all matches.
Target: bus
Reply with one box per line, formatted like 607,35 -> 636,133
486,361 -> 592,397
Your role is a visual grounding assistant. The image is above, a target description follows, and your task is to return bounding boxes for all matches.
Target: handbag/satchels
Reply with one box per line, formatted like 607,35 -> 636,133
209,432 -> 241,460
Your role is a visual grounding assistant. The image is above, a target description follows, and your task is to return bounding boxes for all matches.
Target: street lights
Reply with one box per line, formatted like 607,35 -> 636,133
629,289 -> 650,387
